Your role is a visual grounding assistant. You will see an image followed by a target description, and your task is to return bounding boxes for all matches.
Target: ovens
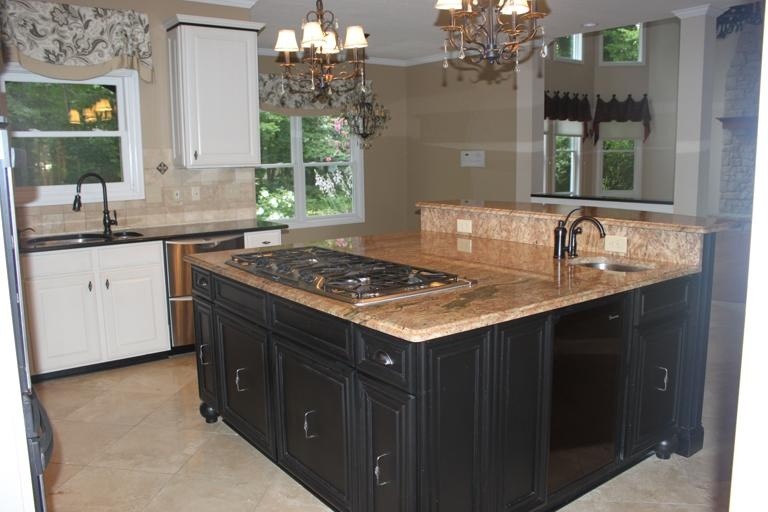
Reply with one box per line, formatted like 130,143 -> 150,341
165,237 -> 246,348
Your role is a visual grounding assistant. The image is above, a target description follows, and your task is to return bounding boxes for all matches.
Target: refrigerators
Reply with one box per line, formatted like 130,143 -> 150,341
0,118 -> 53,512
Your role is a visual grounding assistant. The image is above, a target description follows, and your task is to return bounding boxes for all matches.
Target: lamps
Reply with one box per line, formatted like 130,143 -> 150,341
63,84 -> 114,125
273,0 -> 370,97
337,33 -> 393,154
433,1 -> 553,73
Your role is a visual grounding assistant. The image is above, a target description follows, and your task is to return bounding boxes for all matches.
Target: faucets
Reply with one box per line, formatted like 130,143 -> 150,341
72,172 -> 118,238
568,215 -> 606,257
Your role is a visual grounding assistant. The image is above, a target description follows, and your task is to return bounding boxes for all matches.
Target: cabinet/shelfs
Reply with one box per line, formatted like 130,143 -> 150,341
161,13 -> 267,171
190,262 -> 222,417
213,267 -> 276,462
19,240 -> 173,385
271,284 -> 353,510
353,310 -> 421,512
629,271 -> 697,466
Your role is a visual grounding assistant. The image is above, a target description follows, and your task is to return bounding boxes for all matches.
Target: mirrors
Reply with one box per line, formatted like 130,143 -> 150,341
543,17 -> 681,201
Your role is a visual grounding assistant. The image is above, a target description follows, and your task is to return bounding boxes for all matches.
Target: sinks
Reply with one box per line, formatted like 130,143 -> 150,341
27,231 -> 144,243
569,255 -> 657,273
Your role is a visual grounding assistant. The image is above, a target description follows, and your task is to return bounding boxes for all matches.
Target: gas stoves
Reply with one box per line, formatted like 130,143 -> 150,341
227,244 -> 469,306
